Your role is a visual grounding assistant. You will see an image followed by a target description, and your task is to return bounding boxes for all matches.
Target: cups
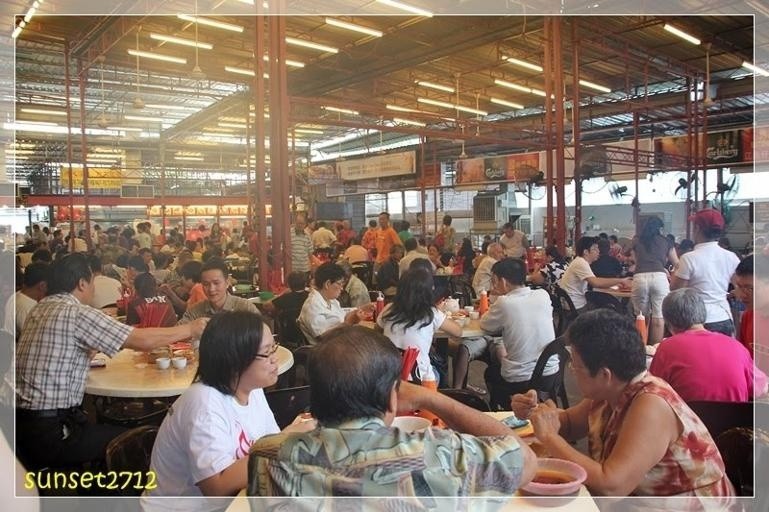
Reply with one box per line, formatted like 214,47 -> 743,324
174,357 -> 187,369
157,357 -> 170,370
437,258 -> 454,275
457,305 -> 480,326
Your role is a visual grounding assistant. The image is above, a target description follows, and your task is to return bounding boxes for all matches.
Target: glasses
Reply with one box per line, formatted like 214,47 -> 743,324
253,343 -> 278,357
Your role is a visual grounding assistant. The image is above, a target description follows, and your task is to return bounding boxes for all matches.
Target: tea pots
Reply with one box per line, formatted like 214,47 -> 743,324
441,295 -> 461,314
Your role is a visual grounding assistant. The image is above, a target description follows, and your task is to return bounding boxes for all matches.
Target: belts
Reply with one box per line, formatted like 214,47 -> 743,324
15,410 -> 70,418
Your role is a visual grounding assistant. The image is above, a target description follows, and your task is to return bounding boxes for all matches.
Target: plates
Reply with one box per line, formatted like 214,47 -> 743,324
148,341 -> 195,364
520,456 -> 587,495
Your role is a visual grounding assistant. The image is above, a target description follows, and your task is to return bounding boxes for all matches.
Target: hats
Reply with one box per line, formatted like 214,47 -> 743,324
688,209 -> 725,229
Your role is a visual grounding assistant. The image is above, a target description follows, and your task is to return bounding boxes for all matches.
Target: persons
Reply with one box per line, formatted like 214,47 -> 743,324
0,202 -> 769,508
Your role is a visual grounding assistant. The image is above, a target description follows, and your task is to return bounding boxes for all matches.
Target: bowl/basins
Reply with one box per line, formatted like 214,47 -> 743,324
393,416 -> 430,433
258,291 -> 273,300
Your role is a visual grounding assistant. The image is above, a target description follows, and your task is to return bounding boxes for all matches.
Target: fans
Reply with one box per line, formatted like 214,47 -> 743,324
671,167 -> 698,198
704,172 -> 743,207
606,180 -> 635,202
514,164 -> 549,200
574,145 -> 611,194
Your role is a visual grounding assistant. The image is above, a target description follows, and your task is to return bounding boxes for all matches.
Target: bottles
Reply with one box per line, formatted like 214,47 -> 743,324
479,289 -> 489,316
634,310 -> 649,347
116,288 -> 130,317
416,362 -> 444,430
375,291 -> 385,318
